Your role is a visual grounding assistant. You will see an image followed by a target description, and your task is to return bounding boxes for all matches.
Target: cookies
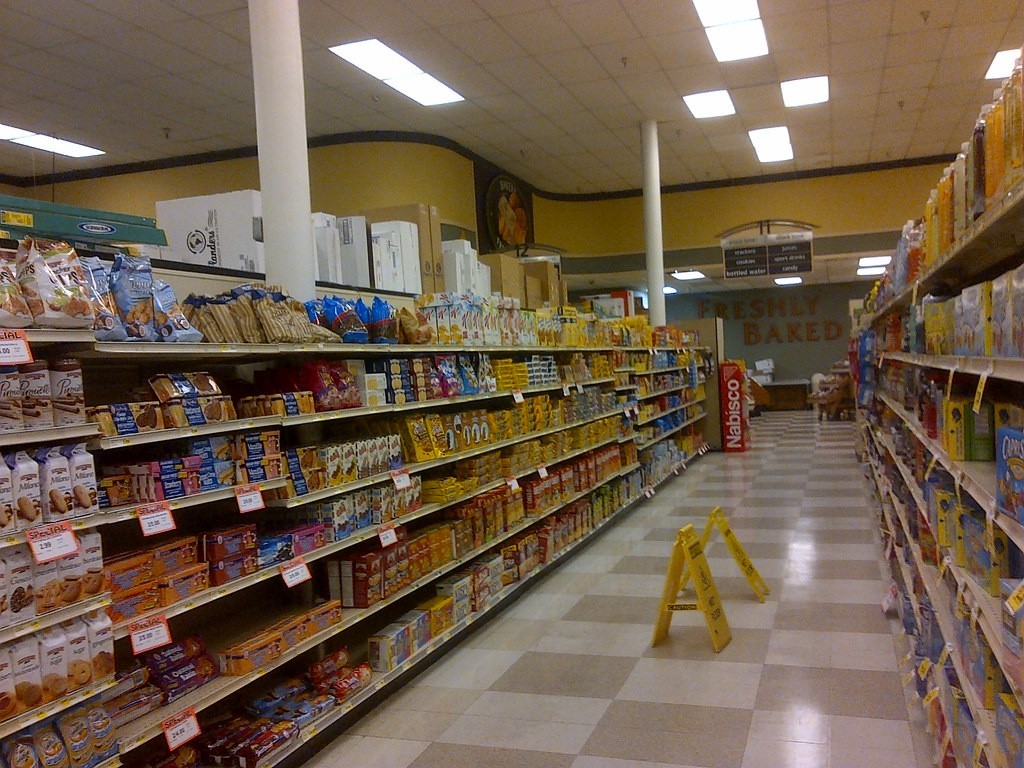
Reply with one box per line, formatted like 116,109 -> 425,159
301,449 -> 320,490
0,486 -> 115,715
6,286 -> 191,337
136,372 -> 221,428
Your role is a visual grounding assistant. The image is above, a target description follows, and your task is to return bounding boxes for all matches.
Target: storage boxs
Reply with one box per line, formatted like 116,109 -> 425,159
915,263 -> 1024,768
86,190 -> 643,725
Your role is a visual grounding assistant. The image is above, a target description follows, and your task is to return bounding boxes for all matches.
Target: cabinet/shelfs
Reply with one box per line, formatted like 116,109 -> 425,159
0,327 -> 711,768
721,363 -> 751,453
807,369 -> 849,421
856,179 -> 1024,768
